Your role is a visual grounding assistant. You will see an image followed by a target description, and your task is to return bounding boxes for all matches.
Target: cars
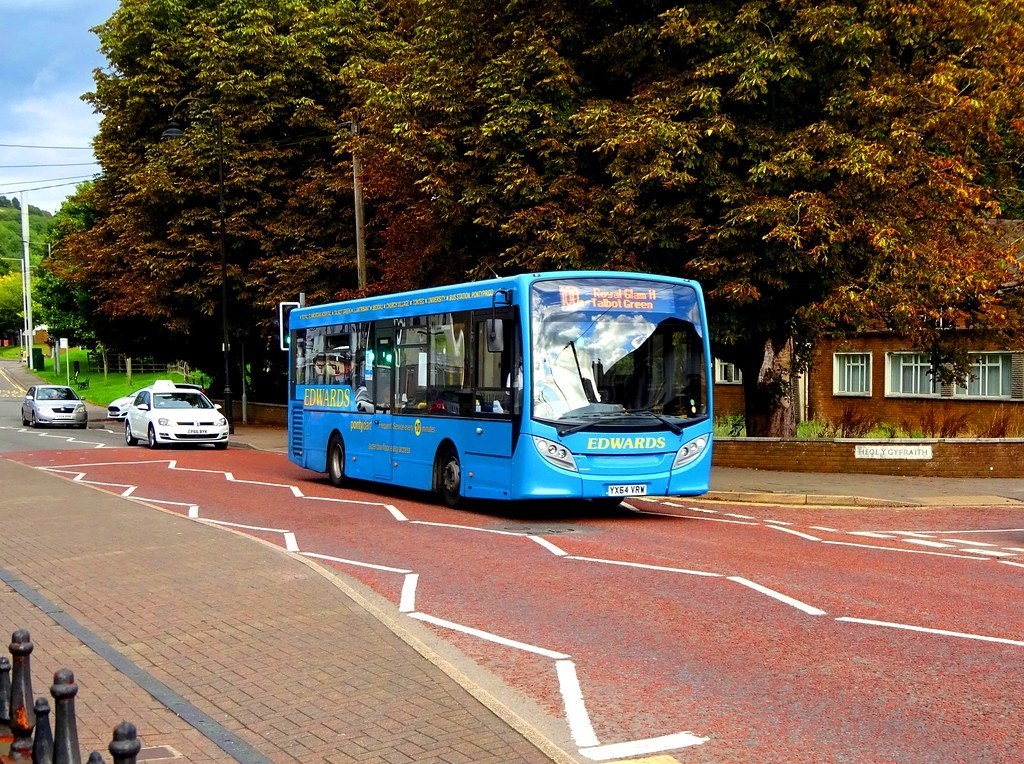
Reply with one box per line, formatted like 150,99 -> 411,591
22,386 -> 87,430
125,388 -> 229,450
107,383 -> 206,422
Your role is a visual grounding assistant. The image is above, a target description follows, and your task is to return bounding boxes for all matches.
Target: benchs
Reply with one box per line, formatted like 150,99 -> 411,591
70,370 -> 90,391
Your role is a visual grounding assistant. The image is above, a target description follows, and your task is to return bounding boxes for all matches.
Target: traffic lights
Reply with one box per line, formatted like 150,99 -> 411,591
280,302 -> 299,351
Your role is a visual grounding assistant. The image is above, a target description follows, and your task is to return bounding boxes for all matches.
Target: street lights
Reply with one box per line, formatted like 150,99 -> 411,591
1,258 -> 29,366
19,241 -> 61,376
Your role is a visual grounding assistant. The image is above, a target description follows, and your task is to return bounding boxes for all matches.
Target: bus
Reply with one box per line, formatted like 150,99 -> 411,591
288,269 -> 716,509
